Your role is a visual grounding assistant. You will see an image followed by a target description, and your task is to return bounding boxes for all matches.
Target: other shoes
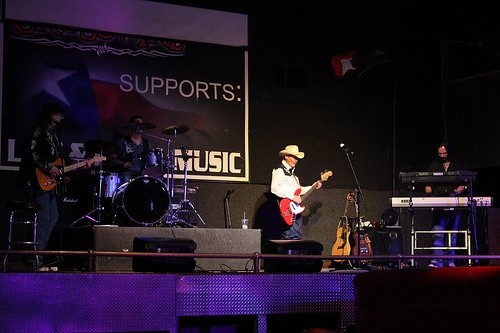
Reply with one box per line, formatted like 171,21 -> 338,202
449,263 -> 455,267
429,261 -> 443,268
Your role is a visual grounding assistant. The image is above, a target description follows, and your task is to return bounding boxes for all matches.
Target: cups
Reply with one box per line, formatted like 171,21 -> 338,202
241,219 -> 250,229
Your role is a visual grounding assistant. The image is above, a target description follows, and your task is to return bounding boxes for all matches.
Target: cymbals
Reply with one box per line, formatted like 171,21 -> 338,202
90,161 -> 131,172
120,122 -> 156,130
174,154 -> 199,160
84,139 -> 121,156
162,125 -> 190,135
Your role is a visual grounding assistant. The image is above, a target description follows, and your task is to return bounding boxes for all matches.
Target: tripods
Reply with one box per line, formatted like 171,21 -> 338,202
167,129 -> 206,229
69,145 -> 120,226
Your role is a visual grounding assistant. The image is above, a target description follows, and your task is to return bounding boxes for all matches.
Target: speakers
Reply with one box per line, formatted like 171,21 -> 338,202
133,237 -> 197,273
363,226 -> 404,266
263,240 -> 324,273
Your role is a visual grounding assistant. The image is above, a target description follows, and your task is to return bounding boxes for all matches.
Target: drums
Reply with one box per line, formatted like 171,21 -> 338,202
140,149 -> 167,178
110,175 -> 170,225
101,173 -> 122,199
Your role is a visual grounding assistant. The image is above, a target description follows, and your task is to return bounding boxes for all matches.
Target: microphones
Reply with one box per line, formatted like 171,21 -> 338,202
340,144 -> 353,154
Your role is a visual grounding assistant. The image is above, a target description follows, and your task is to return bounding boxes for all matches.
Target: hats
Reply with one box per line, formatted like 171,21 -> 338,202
37,102 -> 67,115
279,144 -> 305,159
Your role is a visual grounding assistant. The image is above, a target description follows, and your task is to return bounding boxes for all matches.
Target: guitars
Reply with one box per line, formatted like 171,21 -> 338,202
331,48 -> 386,76
277,170 -> 333,226
352,192 -> 371,263
330,194 -> 352,262
36,155 -> 106,191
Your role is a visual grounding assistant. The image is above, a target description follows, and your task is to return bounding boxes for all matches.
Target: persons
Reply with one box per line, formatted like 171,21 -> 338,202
270,145 -> 322,240
20,101 -> 92,272
108,116 -> 150,168
424,142 -> 470,268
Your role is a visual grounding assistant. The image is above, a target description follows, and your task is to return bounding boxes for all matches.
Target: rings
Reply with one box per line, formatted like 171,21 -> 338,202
54,174 -> 55,175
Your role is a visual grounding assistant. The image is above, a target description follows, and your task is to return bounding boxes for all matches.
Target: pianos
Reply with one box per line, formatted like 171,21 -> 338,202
389,170 -> 493,267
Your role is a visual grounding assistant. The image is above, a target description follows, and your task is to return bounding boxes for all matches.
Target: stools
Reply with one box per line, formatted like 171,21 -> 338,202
3,200 -> 43,272
